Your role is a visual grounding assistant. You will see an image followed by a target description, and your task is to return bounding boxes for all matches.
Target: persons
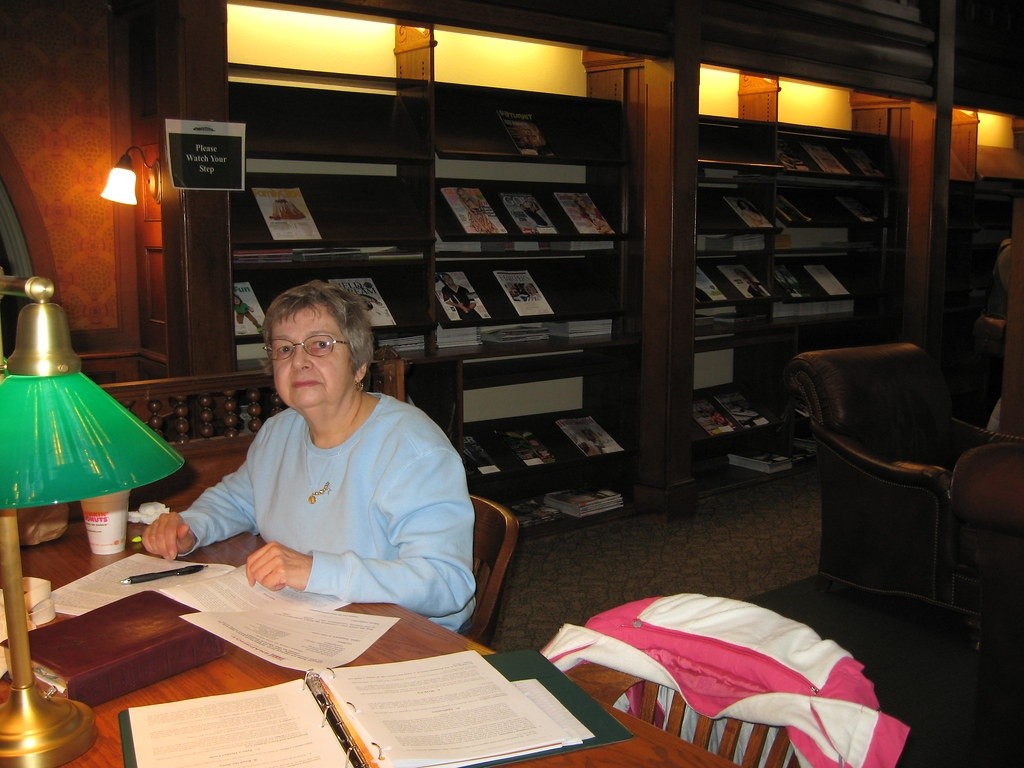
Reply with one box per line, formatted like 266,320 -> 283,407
516,196 -> 546,226
524,284 -> 539,301
442,273 -> 482,319
141,283 -> 478,634
738,197 -> 765,227
975,238 -> 1012,400
459,189 -> 498,232
575,193 -> 610,233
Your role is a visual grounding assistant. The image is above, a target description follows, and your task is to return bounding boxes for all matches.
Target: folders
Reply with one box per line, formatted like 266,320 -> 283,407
118,648 -> 635,768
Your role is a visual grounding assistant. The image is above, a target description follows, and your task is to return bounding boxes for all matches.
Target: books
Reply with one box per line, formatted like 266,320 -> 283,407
497,106 -> 552,158
433,187 -> 614,316
461,416 -> 625,529
693,389 -> 771,437
777,133 -> 883,178
435,318 -> 612,349
252,187 -> 323,239
229,278 -> 425,350
118,649 -> 635,768
697,186 -> 877,313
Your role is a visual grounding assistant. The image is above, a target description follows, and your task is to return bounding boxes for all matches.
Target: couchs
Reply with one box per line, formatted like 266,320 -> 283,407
782,344 -> 1021,639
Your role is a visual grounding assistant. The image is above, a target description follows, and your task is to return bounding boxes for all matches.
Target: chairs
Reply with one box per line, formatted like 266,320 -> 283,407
458,496 -> 520,645
556,593 -> 878,768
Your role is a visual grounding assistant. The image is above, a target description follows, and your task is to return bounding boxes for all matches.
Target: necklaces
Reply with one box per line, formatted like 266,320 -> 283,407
306,432 -> 348,504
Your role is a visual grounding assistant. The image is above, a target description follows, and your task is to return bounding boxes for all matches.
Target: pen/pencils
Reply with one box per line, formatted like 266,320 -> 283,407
120,565 -> 206,585
131,535 -> 142,543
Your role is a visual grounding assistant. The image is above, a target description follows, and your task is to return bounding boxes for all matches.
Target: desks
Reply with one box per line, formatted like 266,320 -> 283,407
0,510 -> 740,768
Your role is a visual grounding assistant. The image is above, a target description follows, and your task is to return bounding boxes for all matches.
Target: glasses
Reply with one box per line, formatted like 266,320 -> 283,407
262,335 -> 351,361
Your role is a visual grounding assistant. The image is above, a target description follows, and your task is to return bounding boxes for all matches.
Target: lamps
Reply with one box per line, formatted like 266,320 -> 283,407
100,146 -> 162,205
1,266 -> 184,767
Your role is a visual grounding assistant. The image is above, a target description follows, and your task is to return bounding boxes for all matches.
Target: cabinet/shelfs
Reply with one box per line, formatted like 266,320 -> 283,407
665,0 -> 946,515
178,1 -> 664,526
929,2 -> 1024,395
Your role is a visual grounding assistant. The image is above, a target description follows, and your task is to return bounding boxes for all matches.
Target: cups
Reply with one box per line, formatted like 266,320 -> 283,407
80,489 -> 130,555
